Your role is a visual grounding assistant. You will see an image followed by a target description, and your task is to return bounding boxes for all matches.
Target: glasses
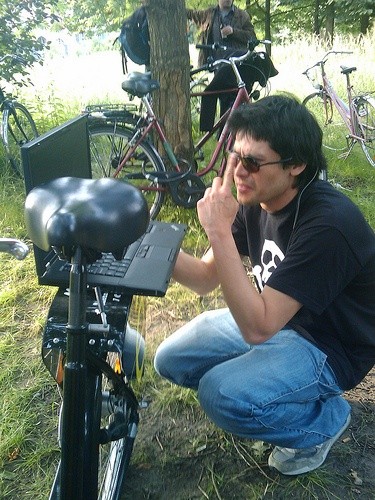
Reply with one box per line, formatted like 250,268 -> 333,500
226,148 -> 295,173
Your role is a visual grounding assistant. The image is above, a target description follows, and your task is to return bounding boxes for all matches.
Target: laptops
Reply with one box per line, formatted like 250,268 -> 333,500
20,112 -> 188,298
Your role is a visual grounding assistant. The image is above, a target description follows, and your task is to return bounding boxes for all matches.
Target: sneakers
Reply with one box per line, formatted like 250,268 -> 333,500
251,412 -> 351,476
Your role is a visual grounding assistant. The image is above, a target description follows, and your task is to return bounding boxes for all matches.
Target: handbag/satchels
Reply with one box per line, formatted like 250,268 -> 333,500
245,52 -> 279,81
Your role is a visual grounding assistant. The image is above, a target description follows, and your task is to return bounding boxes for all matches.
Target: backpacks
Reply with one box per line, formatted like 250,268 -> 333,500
112,6 -> 149,74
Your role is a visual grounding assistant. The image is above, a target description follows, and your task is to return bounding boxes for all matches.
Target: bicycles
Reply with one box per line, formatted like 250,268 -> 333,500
0,53 -> 39,180
83,38 -> 273,222
301,51 -> 375,168
189,63 -> 210,120
125,43 -> 249,82
0,174 -> 147,496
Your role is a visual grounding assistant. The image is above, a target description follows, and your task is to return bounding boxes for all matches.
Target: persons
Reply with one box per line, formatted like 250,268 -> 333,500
141,3 -> 152,102
154,95 -> 374,475
185,0 -> 257,73
